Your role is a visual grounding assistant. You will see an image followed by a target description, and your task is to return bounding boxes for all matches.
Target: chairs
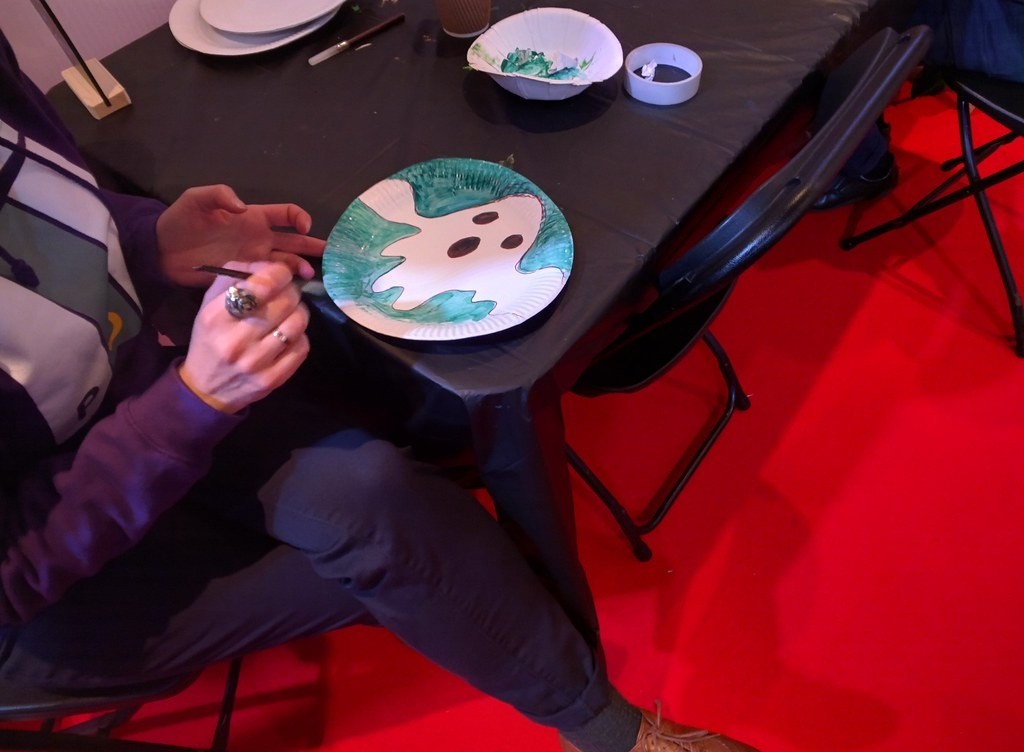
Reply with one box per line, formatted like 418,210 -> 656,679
0,657 -> 278,752
564,25 -> 933,562
839,71 -> 1024,356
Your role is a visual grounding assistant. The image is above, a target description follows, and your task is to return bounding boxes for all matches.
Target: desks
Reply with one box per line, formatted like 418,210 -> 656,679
46,0 -> 877,673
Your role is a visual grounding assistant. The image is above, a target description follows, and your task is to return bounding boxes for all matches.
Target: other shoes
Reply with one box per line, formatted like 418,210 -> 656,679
807,151 -> 900,210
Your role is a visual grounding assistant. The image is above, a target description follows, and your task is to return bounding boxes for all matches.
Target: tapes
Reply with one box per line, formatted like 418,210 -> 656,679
624,42 -> 703,108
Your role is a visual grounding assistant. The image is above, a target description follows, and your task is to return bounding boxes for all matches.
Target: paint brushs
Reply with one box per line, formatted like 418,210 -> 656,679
192,264 -> 327,296
308,12 -> 407,69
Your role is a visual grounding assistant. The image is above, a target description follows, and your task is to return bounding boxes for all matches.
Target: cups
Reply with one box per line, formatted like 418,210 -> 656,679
436,0 -> 493,39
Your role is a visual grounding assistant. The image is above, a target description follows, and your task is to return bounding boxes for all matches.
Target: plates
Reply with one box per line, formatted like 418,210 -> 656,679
168,0 -> 346,57
321,156 -> 576,341
199,0 -> 348,34
465,7 -> 625,100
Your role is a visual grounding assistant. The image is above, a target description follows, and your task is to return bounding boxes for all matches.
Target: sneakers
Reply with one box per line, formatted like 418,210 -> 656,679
558,698 -> 760,752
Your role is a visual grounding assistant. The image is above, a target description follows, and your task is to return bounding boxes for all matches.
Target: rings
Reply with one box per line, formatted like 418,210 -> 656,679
272,329 -> 290,346
225,285 -> 260,319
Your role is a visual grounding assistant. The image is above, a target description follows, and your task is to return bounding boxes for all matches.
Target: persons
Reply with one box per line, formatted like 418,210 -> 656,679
813,2 -> 1023,215
0,18 -> 764,750
